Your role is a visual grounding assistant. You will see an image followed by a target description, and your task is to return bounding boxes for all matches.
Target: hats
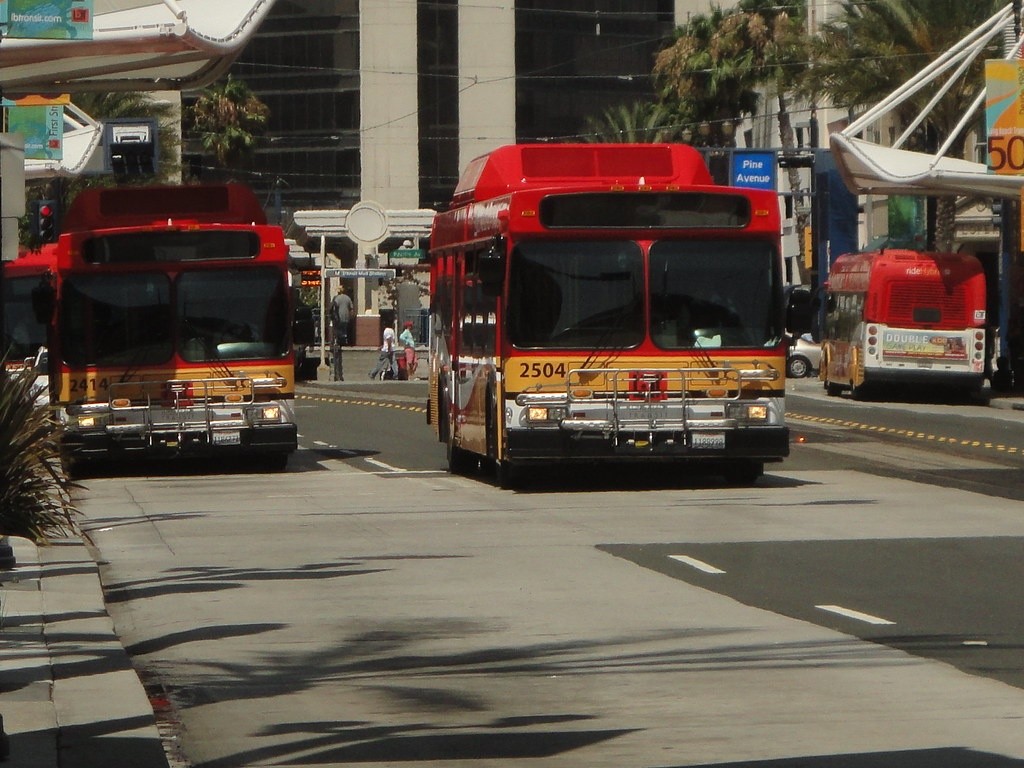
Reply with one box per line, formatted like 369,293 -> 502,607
404,321 -> 413,326
335,285 -> 345,292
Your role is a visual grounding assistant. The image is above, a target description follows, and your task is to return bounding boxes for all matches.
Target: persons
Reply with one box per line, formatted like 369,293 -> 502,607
328,286 -> 354,347
399,320 -> 418,381
367,320 -> 399,381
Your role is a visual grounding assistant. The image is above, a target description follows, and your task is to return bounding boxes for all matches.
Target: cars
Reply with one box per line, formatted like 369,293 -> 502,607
783,329 -> 821,380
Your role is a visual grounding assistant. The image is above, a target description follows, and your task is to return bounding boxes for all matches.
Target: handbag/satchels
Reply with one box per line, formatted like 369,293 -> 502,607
380,369 -> 393,380
395,350 -> 405,359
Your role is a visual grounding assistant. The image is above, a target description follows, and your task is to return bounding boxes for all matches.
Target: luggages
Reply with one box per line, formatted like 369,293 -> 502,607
398,357 -> 407,380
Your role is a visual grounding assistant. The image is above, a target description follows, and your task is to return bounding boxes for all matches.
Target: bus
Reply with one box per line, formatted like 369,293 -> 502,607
428,143 -> 817,492
290,258 -> 335,382
0,247 -> 57,429
817,246 -> 992,406
47,183 -> 301,470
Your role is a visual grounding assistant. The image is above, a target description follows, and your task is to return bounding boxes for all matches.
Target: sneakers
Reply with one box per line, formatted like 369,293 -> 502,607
368,372 -> 375,381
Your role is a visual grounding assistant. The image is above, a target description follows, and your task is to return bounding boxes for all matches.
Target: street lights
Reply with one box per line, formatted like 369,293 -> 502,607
386,239 -> 413,267
